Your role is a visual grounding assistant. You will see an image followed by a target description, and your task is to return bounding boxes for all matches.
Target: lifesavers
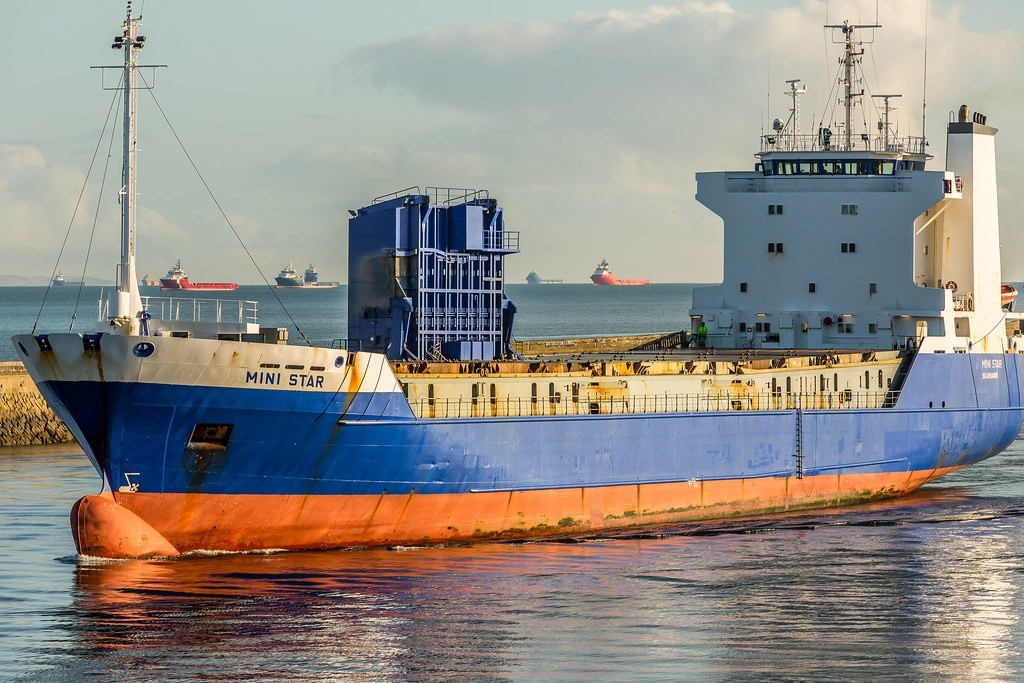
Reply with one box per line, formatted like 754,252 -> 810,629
946,282 -> 958,292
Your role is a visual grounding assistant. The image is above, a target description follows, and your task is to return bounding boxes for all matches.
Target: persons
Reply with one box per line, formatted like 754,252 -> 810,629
697,321 -> 708,350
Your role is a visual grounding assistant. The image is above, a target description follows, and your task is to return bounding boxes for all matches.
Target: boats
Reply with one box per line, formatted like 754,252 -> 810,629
274,260 -> 339,289
141,273 -> 161,287
159,259 -> 240,291
10,0 -> 1024,561
590,257 -> 650,286
51,271 -> 86,288
